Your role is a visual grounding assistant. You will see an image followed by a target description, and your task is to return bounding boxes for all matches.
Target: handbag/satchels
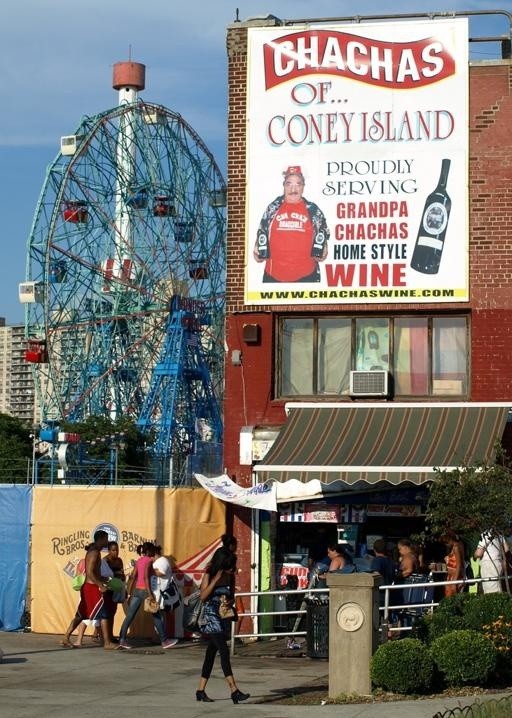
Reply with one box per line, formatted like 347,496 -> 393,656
143,596 -> 159,613
182,590 -> 207,632
159,577 -> 183,613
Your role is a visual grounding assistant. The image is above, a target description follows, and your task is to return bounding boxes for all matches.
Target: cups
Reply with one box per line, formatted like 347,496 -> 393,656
430,563 -> 447,572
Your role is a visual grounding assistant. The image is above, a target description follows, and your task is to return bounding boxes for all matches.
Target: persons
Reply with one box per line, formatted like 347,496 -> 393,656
63,528 -> 180,650
253,166 -> 329,282
285,520 -> 512,660
191,532 -> 250,703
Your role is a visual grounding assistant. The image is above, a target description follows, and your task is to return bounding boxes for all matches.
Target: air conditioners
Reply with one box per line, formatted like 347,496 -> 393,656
349,370 -> 389,397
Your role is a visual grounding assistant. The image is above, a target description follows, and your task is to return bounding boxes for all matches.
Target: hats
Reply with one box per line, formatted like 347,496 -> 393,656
287,166 -> 303,174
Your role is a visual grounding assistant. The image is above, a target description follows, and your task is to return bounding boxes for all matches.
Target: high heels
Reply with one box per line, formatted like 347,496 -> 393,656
231,688 -> 250,705
195,689 -> 216,702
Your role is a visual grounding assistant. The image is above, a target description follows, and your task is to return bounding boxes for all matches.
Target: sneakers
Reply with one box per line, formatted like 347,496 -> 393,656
118,641 -> 134,650
162,637 -> 178,650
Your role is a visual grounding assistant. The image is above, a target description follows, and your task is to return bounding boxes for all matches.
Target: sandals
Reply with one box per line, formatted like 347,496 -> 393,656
104,643 -> 123,650
59,640 -> 78,649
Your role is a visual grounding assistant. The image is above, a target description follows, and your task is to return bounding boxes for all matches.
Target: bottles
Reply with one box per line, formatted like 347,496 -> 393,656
257,218 -> 269,259
311,224 -> 325,258
409,158 -> 452,276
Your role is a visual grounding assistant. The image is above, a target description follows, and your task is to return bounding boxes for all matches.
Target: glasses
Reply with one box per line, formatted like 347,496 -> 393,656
286,183 -> 304,189
